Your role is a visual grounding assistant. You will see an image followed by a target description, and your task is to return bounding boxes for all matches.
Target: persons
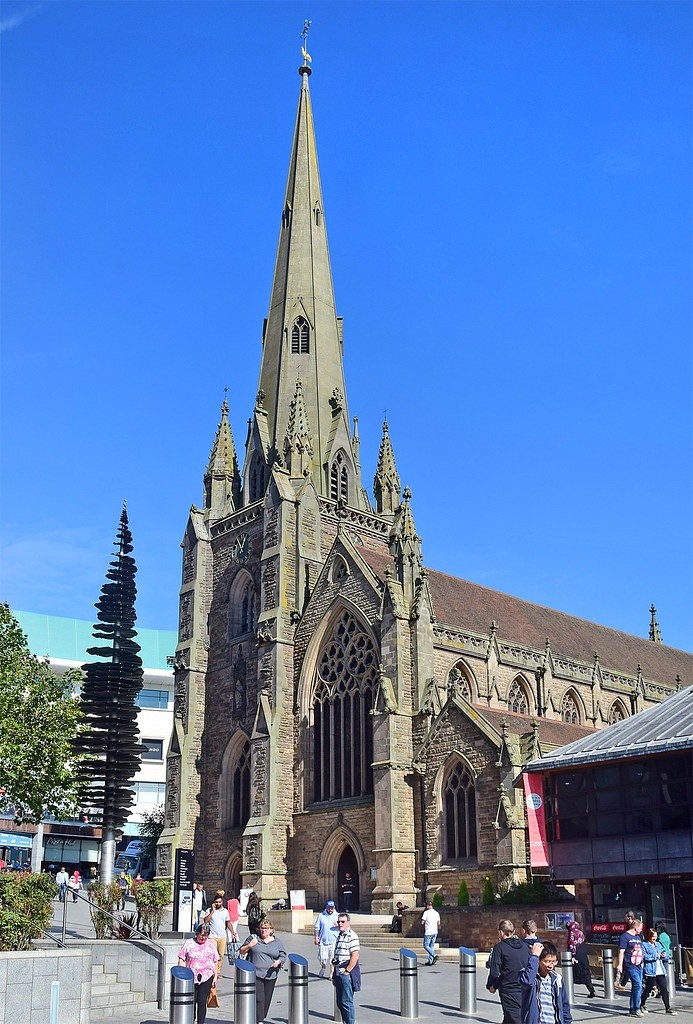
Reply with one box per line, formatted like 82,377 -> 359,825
178,924 -> 220,1024
421,901 -> 441,966
0,859 -> 32,872
69,871 -> 84,903
485,910 -> 678,1024
341,873 -> 354,913
315,900 -> 340,981
56,867 -> 69,902
235,919 -> 287,1024
246,892 -> 263,936
389,901 -> 409,932
131,873 -> 144,907
115,872 -> 128,911
203,895 -> 237,980
193,883 -> 240,942
331,914 -> 361,1024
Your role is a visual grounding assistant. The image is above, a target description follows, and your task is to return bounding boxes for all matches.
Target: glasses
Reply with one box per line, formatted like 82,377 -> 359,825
337,921 -> 348,923
540,959 -> 558,967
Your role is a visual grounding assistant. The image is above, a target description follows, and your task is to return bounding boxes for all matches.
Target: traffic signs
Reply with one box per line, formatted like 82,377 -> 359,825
177,848 -> 195,890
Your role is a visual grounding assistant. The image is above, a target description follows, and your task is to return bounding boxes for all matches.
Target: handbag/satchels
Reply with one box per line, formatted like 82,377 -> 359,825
246,955 -> 252,962
207,988 -> 220,1008
227,937 -> 245,965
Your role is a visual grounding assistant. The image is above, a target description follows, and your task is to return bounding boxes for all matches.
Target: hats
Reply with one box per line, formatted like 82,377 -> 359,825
327,901 -> 336,907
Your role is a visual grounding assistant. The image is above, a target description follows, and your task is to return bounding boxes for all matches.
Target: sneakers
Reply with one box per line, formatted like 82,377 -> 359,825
640,1005 -> 648,1014
319,969 -> 325,978
635,1010 -> 644,1018
630,1011 -> 636,1016
666,1009 -> 677,1016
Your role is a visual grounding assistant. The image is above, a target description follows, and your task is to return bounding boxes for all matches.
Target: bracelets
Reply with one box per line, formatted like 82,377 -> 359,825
345,969 -> 351,973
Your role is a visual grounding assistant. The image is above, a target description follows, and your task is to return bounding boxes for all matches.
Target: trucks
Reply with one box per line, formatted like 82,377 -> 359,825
115,840 -> 156,882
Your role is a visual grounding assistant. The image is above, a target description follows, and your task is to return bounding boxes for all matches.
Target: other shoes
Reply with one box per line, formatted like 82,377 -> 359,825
615,984 -> 625,991
587,992 -> 595,998
425,962 -> 433,966
432,955 -> 439,965
